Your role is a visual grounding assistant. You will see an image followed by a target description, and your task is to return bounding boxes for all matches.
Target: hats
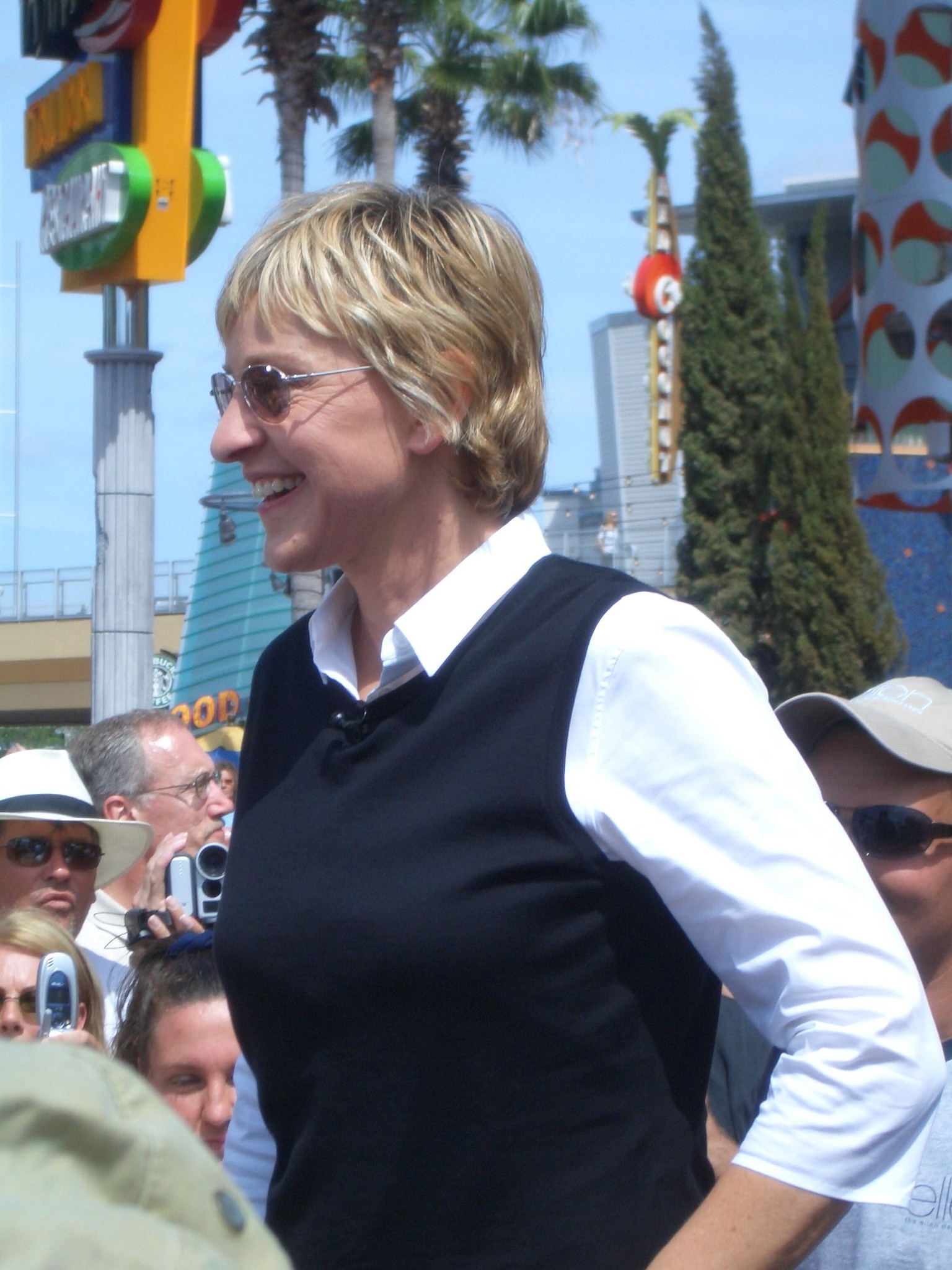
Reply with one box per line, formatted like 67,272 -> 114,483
774,676 -> 952,777
0,749 -> 153,891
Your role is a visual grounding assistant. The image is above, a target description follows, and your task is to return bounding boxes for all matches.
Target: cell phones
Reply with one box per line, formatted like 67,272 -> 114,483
31,949 -> 78,1039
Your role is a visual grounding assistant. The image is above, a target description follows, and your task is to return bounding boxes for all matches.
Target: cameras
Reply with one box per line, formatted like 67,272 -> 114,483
122,841 -> 228,948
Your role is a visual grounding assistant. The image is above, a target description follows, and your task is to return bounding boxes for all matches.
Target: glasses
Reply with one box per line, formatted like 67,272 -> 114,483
210,364 -> 376,425
823,805 -> 952,860
0,987 -> 37,1012
0,835 -> 105,870
141,769 -> 221,800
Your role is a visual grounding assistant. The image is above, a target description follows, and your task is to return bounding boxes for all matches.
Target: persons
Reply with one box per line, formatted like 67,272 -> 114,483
0,707 -> 279,1270
203,176 -> 946,1270
706,676 -> 952,1270
